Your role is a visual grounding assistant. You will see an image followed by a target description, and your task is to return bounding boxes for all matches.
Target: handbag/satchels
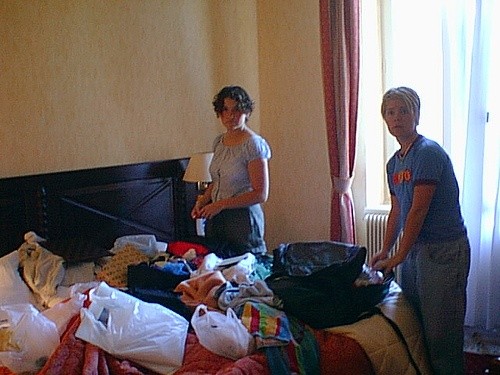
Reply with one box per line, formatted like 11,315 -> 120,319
101,235 -> 259,361
0,251 -> 87,375
74,280 -> 189,375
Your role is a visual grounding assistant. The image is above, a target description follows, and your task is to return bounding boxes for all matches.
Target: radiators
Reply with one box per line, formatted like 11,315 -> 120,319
362,213 -> 404,286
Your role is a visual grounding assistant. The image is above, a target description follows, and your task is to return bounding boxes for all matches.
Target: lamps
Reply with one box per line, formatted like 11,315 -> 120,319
182,151 -> 214,237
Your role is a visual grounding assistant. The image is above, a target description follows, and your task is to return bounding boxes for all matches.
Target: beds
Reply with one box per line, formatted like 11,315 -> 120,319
0,159 -> 433,375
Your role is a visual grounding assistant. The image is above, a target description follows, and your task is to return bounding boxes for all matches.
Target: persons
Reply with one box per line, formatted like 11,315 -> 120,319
370,86 -> 470,375
191,85 -> 272,256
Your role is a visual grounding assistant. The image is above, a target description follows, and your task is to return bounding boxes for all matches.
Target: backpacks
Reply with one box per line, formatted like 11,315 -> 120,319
264,241 -> 396,329
